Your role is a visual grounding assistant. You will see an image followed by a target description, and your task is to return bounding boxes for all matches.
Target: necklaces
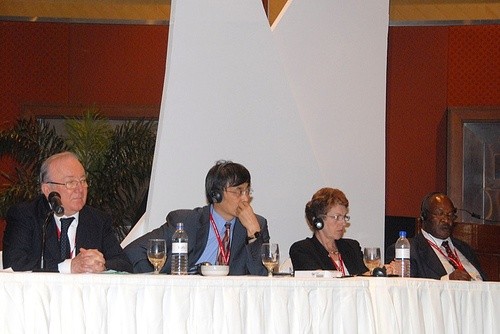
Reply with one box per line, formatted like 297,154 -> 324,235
329,249 -> 338,255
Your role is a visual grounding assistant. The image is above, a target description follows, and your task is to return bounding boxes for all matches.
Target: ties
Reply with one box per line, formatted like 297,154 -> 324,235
217,222 -> 231,266
60,217 -> 76,263
441,241 -> 471,278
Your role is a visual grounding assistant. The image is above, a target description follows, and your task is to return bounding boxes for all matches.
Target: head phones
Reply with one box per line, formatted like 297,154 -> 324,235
418,192 -> 440,223
209,162 -> 232,204
311,202 -> 324,230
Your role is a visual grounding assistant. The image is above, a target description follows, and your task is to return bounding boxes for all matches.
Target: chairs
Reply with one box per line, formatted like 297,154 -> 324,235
384,215 -> 416,264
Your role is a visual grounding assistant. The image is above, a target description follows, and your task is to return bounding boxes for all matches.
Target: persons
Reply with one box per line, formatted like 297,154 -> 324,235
123,159 -> 270,276
3,152 -> 134,273
389,192 -> 488,281
290,188 -> 393,276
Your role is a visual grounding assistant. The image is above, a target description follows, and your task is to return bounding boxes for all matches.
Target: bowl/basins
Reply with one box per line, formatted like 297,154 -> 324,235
200,265 -> 230,276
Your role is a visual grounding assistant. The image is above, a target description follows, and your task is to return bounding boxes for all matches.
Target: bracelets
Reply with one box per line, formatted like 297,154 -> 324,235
248,231 -> 260,240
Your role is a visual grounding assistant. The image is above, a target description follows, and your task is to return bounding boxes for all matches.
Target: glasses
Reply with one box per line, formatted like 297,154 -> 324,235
44,179 -> 92,190
221,188 -> 254,195
424,211 -> 455,219
322,215 -> 350,222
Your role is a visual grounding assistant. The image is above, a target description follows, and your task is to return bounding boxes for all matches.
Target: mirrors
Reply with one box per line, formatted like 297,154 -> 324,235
446,104 -> 500,226
18,103 -> 160,181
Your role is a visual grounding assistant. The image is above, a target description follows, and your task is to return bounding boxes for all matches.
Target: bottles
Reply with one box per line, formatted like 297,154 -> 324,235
393,231 -> 410,277
170,222 -> 188,275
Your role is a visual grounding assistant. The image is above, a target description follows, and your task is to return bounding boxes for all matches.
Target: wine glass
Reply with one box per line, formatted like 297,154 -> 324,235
363,247 -> 381,276
260,243 -> 279,277
147,238 -> 167,274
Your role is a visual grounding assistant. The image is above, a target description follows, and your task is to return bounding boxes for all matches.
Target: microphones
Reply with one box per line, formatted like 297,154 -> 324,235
47,191 -> 63,213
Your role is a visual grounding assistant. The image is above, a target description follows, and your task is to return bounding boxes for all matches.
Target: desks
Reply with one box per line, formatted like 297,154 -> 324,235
0,267 -> 500,334
